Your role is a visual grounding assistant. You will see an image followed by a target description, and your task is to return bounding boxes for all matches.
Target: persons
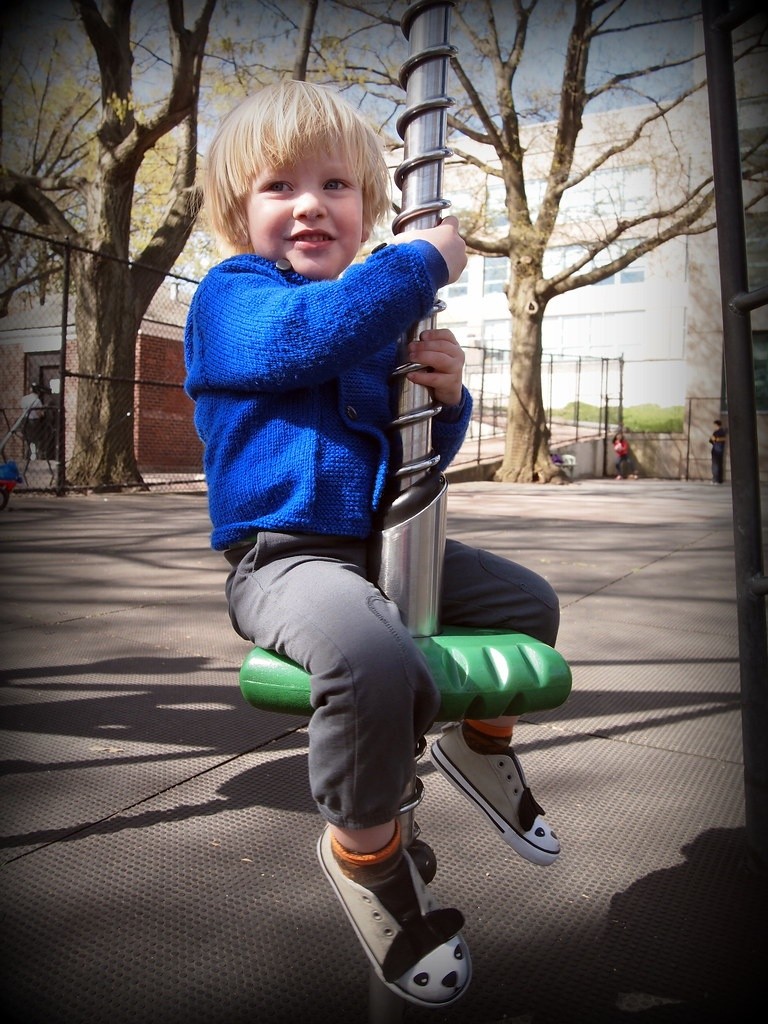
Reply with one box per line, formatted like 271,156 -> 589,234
612,432 -> 638,480
183,80 -> 561,1005
709,420 -> 726,485
18,382 -> 43,461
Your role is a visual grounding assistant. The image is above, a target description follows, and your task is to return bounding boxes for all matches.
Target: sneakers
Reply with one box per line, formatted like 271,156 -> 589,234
428,721 -> 561,870
313,819 -> 475,1007
